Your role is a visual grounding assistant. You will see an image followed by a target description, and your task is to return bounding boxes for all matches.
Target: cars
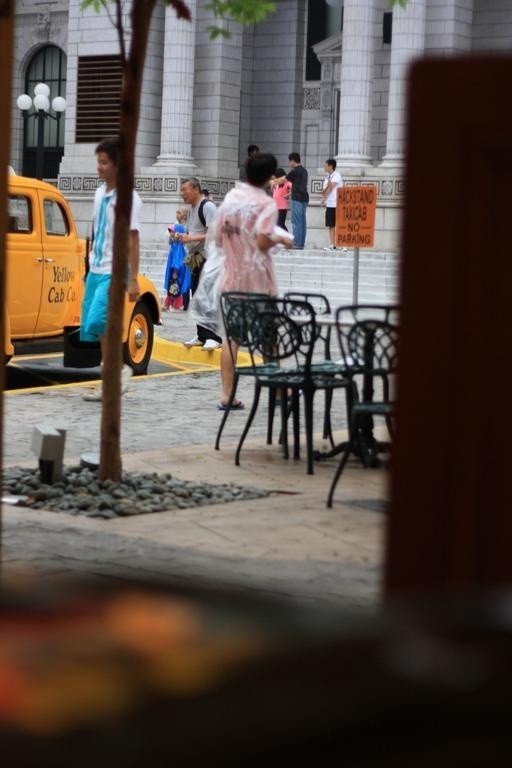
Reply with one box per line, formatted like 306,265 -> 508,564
2,166 -> 162,375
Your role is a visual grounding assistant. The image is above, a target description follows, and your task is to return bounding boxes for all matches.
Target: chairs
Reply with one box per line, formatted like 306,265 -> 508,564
215,290 -> 295,449
235,297 -> 354,474
279,292 -> 346,443
326,303 -> 398,507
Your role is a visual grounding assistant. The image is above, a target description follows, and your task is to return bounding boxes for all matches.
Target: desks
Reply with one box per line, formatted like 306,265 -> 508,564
289,314 -> 398,469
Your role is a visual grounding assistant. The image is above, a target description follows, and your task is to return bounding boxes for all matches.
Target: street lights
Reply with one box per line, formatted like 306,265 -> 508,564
15,82 -> 66,177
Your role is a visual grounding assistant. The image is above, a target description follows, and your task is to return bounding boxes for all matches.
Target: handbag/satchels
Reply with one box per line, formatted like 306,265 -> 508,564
183,254 -> 203,272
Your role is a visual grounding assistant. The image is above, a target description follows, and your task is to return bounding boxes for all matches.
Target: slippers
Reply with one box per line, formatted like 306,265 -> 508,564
217,399 -> 245,409
275,389 -> 303,407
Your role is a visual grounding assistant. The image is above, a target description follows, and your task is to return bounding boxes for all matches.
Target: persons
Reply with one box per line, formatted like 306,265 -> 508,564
161,145 -> 309,412
78,138 -> 142,402
320,159 -> 343,251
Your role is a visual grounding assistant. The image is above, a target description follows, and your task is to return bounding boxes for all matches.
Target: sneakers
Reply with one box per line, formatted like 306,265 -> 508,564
184,335 -> 203,346
120,364 -> 134,397
201,338 -> 223,351
323,245 -> 337,251
82,383 -> 102,402
